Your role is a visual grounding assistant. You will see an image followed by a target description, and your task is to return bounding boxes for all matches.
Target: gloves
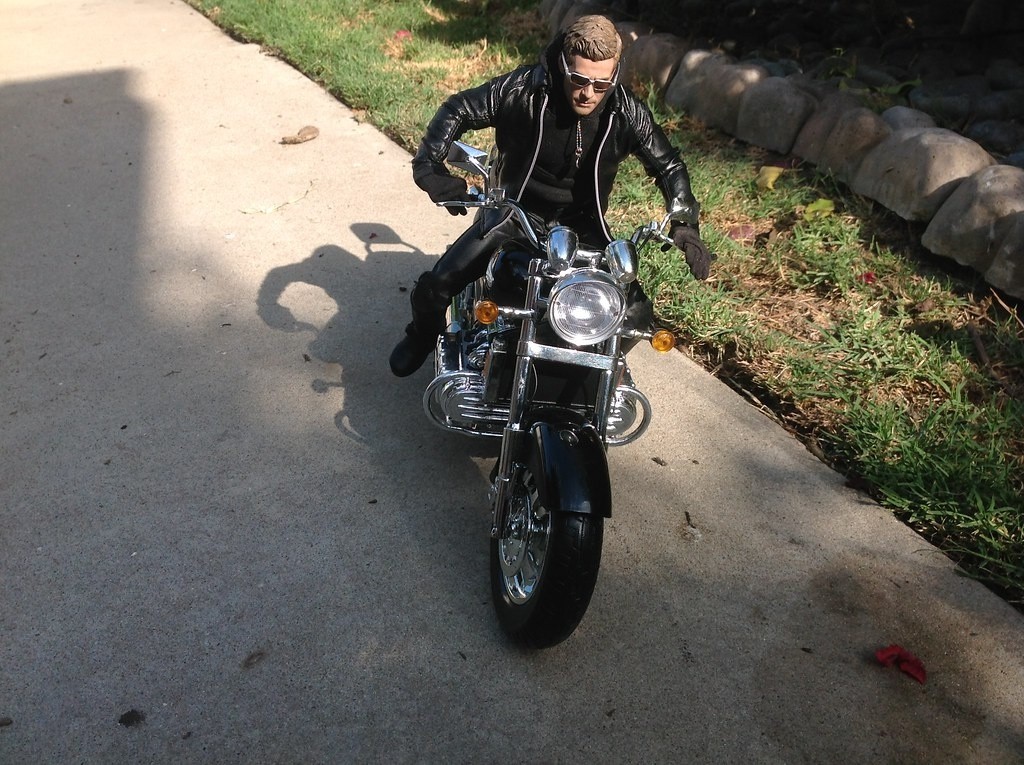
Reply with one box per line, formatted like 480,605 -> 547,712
673,226 -> 710,281
420,173 -> 467,217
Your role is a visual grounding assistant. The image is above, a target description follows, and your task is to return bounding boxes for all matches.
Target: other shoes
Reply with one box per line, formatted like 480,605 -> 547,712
389,316 -> 447,377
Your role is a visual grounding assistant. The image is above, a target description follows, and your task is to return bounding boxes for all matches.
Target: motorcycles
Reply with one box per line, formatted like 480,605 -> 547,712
420,137 -> 717,648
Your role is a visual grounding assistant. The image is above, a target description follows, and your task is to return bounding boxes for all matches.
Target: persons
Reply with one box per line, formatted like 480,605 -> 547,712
390,16 -> 712,377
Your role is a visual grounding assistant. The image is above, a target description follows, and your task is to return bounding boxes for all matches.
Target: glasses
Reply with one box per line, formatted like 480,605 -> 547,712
559,51 -> 620,90
